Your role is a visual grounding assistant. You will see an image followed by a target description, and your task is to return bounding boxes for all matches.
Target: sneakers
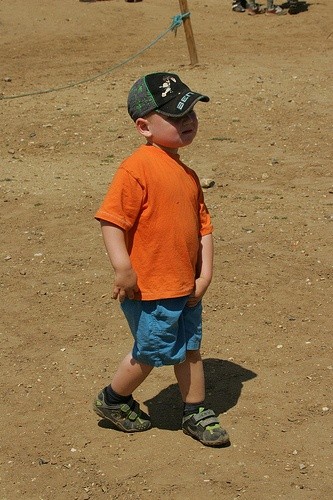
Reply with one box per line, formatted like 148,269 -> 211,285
93,387 -> 152,432
182,407 -> 230,447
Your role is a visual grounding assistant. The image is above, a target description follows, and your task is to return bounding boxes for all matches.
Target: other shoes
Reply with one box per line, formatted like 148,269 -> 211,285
246,5 -> 266,15
231,1 -> 244,12
240,0 -> 250,9
264,6 -> 287,16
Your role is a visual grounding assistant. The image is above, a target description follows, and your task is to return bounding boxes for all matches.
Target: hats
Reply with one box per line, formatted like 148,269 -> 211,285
127,72 -> 209,122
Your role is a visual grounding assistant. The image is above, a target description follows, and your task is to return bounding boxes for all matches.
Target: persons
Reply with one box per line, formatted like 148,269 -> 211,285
93,72 -> 231,446
248,0 -> 275,16
